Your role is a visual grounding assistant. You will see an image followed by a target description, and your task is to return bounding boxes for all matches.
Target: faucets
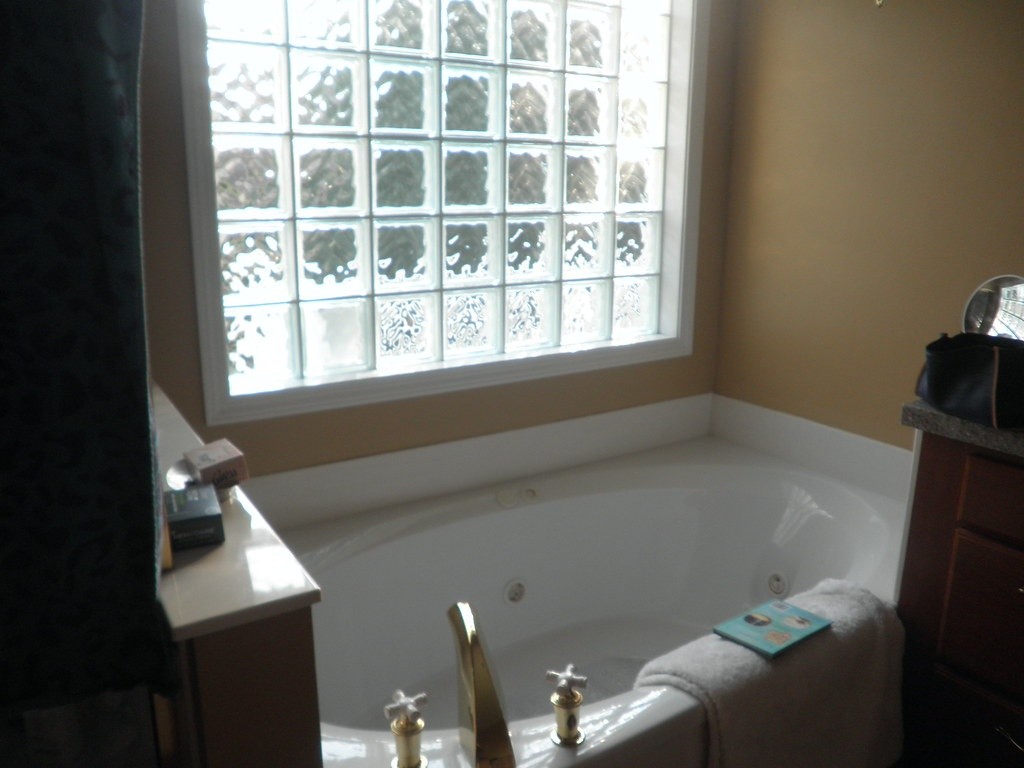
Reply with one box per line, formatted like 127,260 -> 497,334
445,601 -> 517,768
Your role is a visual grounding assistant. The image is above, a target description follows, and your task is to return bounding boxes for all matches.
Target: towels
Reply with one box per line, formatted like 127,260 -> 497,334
632,576 -> 907,768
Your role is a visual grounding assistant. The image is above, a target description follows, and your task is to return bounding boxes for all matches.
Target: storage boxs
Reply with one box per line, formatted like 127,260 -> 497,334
184,437 -> 247,489
163,483 -> 226,551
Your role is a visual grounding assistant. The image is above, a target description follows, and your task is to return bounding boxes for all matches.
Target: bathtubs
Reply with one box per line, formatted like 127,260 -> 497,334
279,438 -> 908,768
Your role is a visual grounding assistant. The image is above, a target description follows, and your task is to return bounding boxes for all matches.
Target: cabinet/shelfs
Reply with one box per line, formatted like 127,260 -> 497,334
892,398 -> 1024,768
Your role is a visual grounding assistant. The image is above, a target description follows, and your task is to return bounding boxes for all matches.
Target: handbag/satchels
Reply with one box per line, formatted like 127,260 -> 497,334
913,332 -> 1024,429
